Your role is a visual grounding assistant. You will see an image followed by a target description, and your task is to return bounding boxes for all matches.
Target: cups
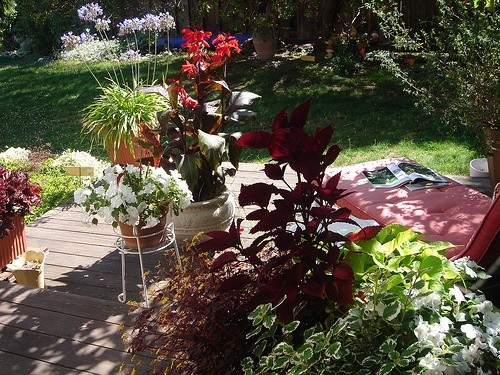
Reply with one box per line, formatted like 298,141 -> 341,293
470,158 -> 489,177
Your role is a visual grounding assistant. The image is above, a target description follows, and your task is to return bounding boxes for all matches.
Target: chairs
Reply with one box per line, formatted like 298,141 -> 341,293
306,155 -> 500,312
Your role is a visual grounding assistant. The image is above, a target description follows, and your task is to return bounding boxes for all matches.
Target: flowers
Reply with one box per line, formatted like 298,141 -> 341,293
401,256 -> 500,375
0,166 -> 42,239
169,27 -> 240,110
73,161 -> 193,231
61,3 -> 176,102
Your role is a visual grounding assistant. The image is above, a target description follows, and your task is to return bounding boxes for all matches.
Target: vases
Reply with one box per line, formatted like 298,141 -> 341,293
0,120 -> 234,289
